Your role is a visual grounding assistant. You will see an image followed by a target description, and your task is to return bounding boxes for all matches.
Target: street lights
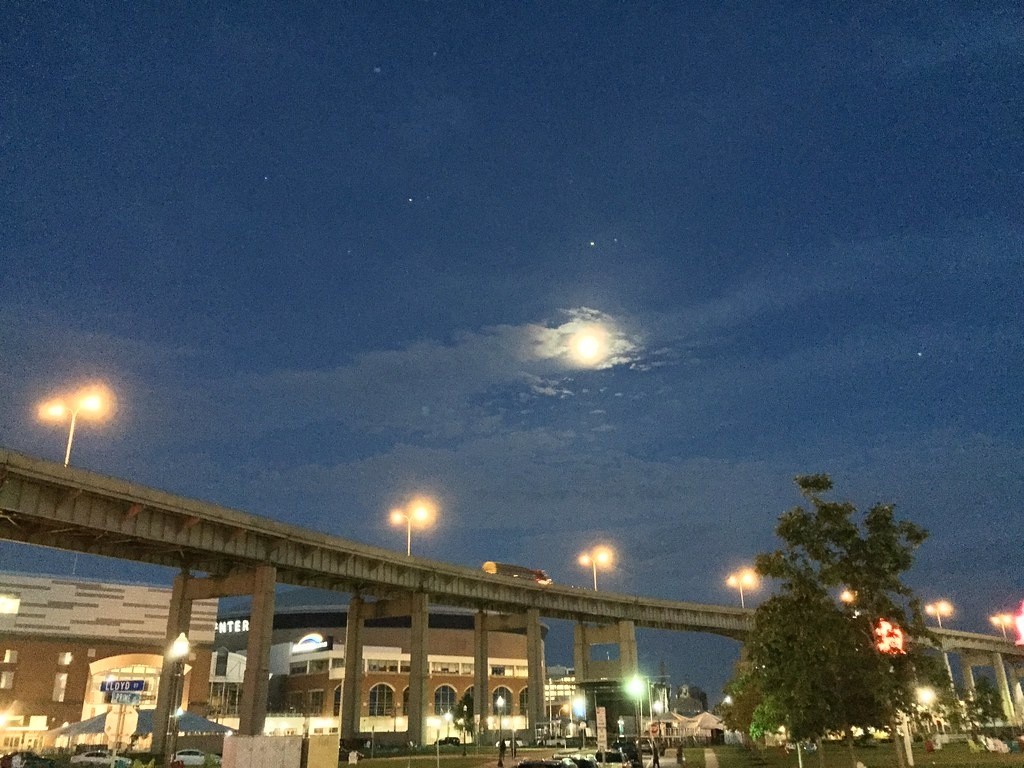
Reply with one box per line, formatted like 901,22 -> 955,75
390,506 -> 435,555
431,720 -> 441,768
578,551 -> 613,593
926,602 -> 952,628
35,391 -> 114,469
444,712 -> 452,744
727,569 -> 760,608
462,705 -> 468,756
170,633 -> 188,763
497,696 -> 506,758
991,614 -> 1011,639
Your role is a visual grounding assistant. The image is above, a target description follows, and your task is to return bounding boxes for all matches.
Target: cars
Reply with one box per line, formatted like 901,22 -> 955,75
538,734 -> 567,747
71,750 -> 132,766
169,749 -> 221,766
513,736 -> 665,767
496,737 -> 522,747
1,751 -> 54,767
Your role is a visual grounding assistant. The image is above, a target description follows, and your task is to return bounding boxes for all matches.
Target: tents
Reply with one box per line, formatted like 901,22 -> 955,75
51,709 -> 238,735
653,711 -> 725,730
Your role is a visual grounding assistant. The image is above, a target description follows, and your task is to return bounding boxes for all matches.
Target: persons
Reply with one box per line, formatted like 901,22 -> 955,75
926,731 -> 942,753
653,748 -> 660,768
348,748 -> 365,765
11,752 -> 26,768
510,738 -> 519,758
498,738 -> 506,760
